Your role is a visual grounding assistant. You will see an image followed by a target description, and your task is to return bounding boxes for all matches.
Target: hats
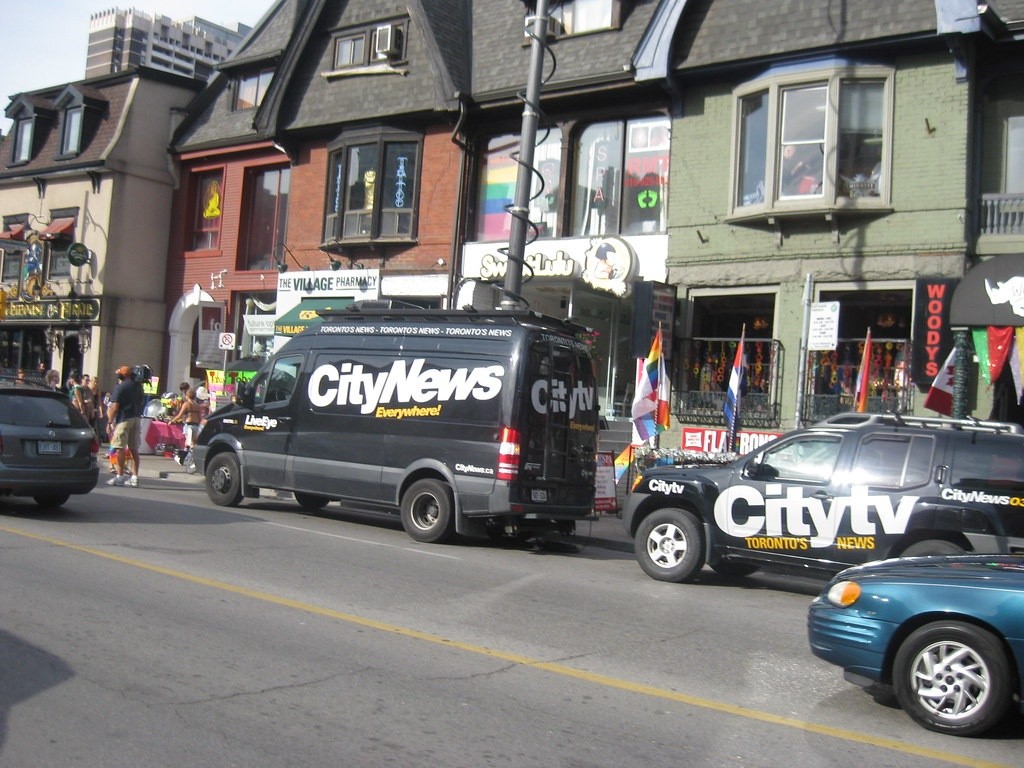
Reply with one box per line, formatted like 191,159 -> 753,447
115,365 -> 132,377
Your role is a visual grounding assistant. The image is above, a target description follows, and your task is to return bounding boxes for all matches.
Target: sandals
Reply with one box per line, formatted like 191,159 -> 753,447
109,467 -> 118,474
123,467 -> 132,475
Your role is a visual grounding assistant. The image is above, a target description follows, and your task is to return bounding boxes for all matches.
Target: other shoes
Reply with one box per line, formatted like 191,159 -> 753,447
102,453 -> 109,459
125,477 -> 138,487
106,475 -> 125,486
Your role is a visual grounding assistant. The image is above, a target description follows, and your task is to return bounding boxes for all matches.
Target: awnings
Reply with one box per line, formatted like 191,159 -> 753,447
39,218 -> 75,241
0,225 -> 26,246
274,299 -> 354,336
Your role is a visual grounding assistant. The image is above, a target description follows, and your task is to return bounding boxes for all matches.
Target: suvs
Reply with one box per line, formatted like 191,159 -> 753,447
0,376 -> 101,504
620,413 -> 1023,585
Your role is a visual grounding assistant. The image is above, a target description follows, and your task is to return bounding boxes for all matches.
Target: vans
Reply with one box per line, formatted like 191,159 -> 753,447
192,300 -> 601,544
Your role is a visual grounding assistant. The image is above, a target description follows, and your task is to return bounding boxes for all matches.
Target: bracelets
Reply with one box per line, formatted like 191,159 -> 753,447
171,420 -> 173,424
108,422 -> 112,424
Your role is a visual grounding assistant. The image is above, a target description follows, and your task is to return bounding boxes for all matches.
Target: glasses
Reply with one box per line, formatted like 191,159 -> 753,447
83,379 -> 91,382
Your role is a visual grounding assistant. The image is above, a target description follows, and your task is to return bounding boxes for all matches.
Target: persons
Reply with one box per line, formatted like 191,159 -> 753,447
168,389 -> 200,472
754,144 -> 810,205
103,366 -> 144,487
15,368 -> 25,383
180,383 -> 190,461
46,370 -> 60,391
60,373 -> 103,445
28,363 -> 46,387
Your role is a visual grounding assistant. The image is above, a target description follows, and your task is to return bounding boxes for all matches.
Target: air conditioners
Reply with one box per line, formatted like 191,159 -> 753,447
376,25 -> 402,57
525,15 -> 560,39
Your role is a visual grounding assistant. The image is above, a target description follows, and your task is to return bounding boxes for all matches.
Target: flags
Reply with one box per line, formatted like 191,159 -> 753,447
723,341 -> 748,430
633,331 -> 670,441
855,331 -> 871,412
925,347 -> 956,417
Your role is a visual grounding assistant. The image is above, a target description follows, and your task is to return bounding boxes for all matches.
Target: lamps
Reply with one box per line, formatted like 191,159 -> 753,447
272,240 -> 310,274
326,238 -> 364,271
44,325 -> 59,353
77,325 -> 91,354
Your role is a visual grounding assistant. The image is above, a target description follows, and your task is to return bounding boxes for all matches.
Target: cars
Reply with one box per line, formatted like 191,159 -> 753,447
807,554 -> 1023,735
